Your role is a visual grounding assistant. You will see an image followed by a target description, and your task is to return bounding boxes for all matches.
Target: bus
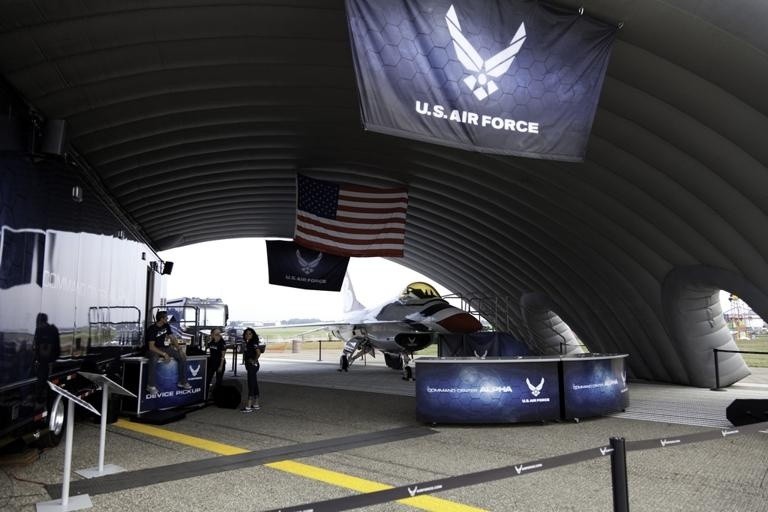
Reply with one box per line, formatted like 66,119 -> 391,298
164,298 -> 228,351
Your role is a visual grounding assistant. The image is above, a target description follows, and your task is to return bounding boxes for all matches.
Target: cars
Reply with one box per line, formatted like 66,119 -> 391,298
225,325 -> 267,355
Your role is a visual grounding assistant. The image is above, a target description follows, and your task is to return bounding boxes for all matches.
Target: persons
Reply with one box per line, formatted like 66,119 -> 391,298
206,329 -> 225,398
240,328 -> 261,412
32,314 -> 61,363
145,311 -> 192,394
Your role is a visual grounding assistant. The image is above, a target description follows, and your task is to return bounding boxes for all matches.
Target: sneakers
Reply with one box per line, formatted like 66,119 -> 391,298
177,381 -> 192,389
145,384 -> 160,395
240,404 -> 260,413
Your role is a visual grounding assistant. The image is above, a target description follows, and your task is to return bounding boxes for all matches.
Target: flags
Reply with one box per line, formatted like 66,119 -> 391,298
293,174 -> 408,257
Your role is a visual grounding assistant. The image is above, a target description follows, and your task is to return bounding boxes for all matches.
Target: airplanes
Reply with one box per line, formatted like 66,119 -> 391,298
236,268 -> 485,383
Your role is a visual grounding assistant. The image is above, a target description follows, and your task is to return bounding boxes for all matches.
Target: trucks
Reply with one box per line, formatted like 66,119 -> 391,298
0,68 -> 175,455
